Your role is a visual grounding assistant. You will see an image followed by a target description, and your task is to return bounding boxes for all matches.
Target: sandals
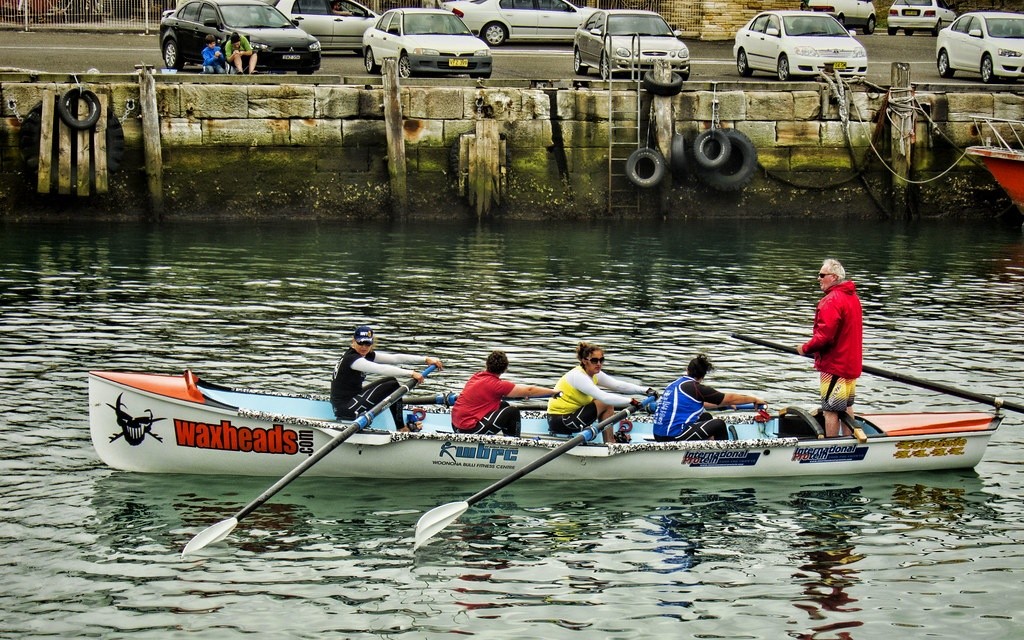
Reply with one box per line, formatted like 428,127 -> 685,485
399,421 -> 422,433
613,432 -> 632,443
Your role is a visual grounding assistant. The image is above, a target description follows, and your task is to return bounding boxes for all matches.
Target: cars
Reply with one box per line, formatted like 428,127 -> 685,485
935,12 -> 1024,84
159,0 -> 321,75
808,0 -> 876,35
362,8 -> 492,78
441,0 -> 601,46
260,0 -> 381,56
886,0 -> 957,37
733,11 -> 868,81
574,9 -> 691,81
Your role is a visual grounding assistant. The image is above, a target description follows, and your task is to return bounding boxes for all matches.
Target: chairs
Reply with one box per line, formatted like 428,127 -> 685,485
223,35 -> 251,75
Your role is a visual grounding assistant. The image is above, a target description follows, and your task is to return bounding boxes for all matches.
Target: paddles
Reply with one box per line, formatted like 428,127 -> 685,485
516,404 -> 768,411
402,391 -> 564,406
413,393 -> 658,554
729,332 -> 1024,416
179,363 -> 440,558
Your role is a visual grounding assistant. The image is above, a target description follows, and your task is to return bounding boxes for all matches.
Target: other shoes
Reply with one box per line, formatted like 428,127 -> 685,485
251,71 -> 259,75
237,71 -> 244,75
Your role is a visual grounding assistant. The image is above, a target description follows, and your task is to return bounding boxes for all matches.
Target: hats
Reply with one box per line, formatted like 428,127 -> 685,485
354,327 -> 374,341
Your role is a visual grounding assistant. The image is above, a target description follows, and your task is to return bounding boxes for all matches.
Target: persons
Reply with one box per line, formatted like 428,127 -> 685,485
546,342 -> 660,442
795,258 -> 864,438
225,32 -> 259,74
330,326 -> 444,432
451,350 -> 562,436
201,34 -> 230,74
653,353 -> 768,441
331,0 -> 353,15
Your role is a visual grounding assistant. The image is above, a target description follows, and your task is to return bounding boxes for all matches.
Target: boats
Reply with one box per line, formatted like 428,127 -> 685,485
87,369 -> 1001,481
965,114 -> 1024,216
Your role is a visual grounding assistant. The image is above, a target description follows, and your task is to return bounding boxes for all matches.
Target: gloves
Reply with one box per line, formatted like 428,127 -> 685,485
630,398 -> 642,411
646,387 -> 659,401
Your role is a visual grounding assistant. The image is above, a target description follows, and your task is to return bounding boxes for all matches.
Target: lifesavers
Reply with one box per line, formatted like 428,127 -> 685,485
691,127 -> 759,196
671,134 -> 691,181
57,87 -> 102,131
694,129 -> 732,170
643,68 -> 682,98
625,147 -> 666,189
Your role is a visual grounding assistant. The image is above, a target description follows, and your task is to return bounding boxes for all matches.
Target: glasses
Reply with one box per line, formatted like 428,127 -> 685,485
818,273 -> 833,278
356,341 -> 373,346
583,356 -> 604,363
